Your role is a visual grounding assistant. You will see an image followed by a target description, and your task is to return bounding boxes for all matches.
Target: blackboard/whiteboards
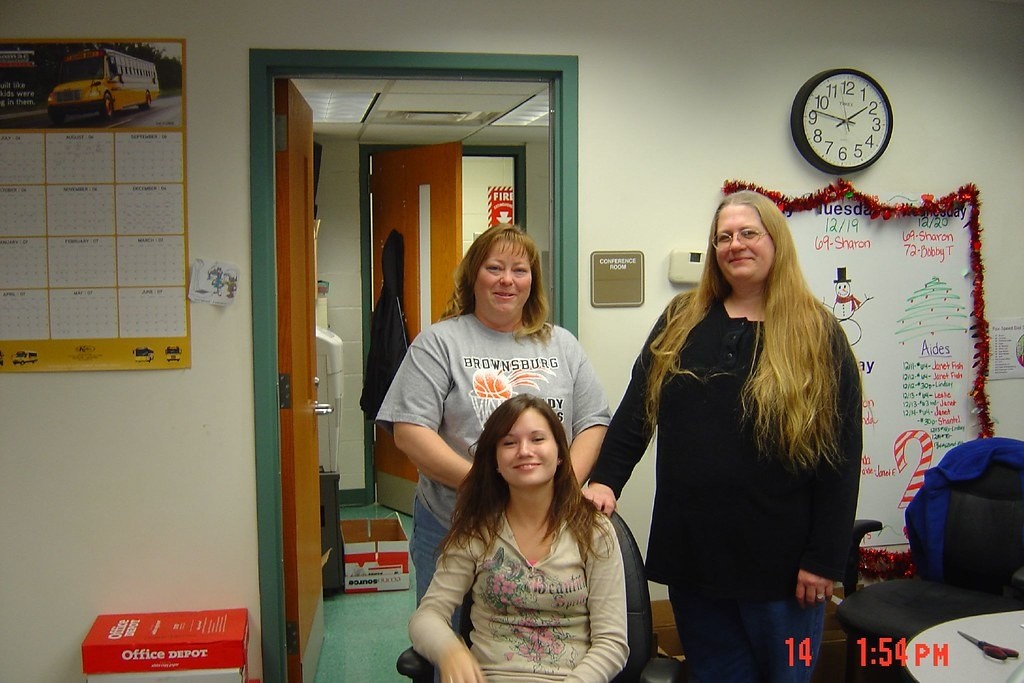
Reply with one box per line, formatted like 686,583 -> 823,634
779,192 -> 984,549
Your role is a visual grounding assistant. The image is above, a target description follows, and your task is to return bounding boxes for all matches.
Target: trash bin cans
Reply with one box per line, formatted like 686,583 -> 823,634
320,465 -> 344,589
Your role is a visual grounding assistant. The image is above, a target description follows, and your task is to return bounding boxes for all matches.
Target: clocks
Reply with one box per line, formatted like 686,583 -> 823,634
790,68 -> 894,176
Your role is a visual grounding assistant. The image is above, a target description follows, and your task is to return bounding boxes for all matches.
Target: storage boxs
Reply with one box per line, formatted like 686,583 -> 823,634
81,608 -> 259,683
339,517 -> 411,594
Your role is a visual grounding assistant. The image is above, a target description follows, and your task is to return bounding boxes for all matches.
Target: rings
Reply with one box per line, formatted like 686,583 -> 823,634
816,594 -> 823,599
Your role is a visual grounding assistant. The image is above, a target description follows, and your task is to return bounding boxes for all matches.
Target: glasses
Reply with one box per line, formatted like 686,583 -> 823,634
712,229 -> 768,250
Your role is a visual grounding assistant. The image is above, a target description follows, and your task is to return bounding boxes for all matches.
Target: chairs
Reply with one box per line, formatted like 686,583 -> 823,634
395,504 -> 682,683
833,437 -> 1024,683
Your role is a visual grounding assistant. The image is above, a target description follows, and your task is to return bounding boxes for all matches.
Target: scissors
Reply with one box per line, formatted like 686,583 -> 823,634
957,630 -> 1019,661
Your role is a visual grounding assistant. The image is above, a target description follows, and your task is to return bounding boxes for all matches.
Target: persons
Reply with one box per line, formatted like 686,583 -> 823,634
408,395 -> 631,683
580,191 -> 864,683
375,223 -> 613,683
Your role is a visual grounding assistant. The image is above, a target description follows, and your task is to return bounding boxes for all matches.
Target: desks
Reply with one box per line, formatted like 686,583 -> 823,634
903,610 -> 1024,683
